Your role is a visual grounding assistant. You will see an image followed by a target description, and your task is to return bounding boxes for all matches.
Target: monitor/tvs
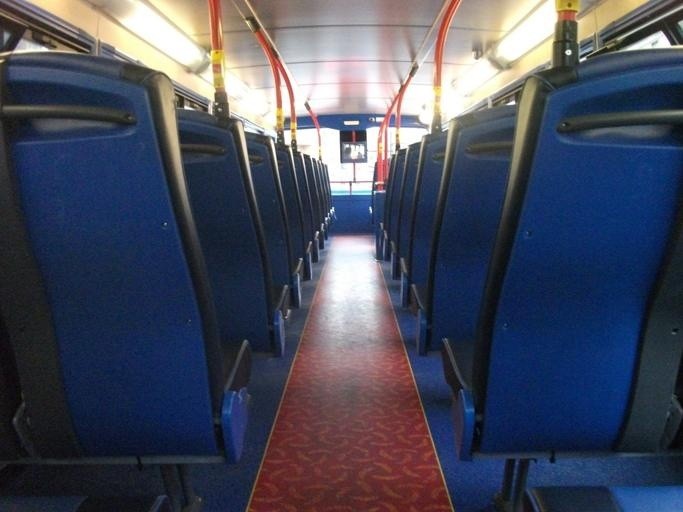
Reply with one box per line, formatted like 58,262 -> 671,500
341,141 -> 367,163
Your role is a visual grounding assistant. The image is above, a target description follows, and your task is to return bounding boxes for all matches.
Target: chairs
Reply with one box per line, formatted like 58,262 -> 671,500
369,48 -> 683,512
0,51 -> 336,511
